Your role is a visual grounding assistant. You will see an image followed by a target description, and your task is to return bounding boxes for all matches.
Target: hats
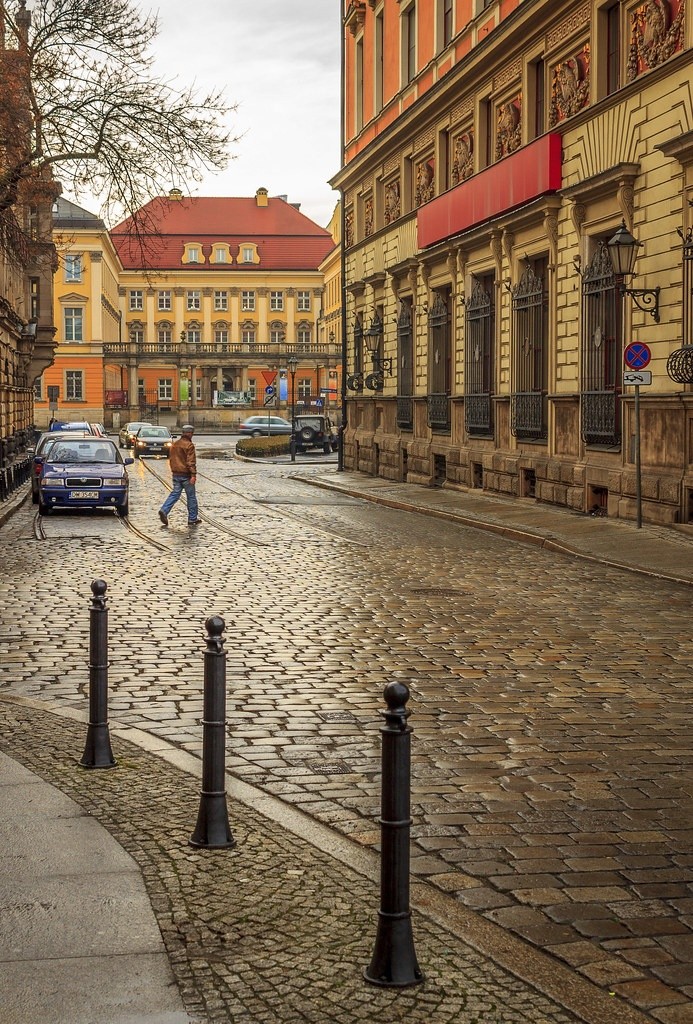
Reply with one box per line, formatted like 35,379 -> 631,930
182,425 -> 194,433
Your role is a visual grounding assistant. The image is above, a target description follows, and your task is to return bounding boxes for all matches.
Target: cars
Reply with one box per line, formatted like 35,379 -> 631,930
27,430 -> 92,504
118,422 -> 152,448
50,420 -> 110,439
239,416 -> 292,438
132,426 -> 178,459
34,436 -> 134,515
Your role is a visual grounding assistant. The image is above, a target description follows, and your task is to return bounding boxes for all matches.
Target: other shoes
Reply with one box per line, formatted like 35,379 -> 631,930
188,519 -> 202,525
159,510 -> 168,524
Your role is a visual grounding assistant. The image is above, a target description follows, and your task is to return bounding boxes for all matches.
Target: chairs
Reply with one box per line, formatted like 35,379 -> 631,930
95,449 -> 109,459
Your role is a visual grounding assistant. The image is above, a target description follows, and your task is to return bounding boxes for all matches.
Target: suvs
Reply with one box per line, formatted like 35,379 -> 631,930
289,414 -> 337,455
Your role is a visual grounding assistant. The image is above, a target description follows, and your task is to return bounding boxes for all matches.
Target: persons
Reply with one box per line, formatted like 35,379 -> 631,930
159,424 -> 202,525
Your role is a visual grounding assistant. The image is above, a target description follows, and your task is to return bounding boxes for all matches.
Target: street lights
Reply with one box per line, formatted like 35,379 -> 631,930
287,354 -> 298,418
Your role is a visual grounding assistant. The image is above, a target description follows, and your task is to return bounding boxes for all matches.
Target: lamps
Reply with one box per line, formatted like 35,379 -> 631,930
363,328 -> 392,375
606,218 -> 661,324
